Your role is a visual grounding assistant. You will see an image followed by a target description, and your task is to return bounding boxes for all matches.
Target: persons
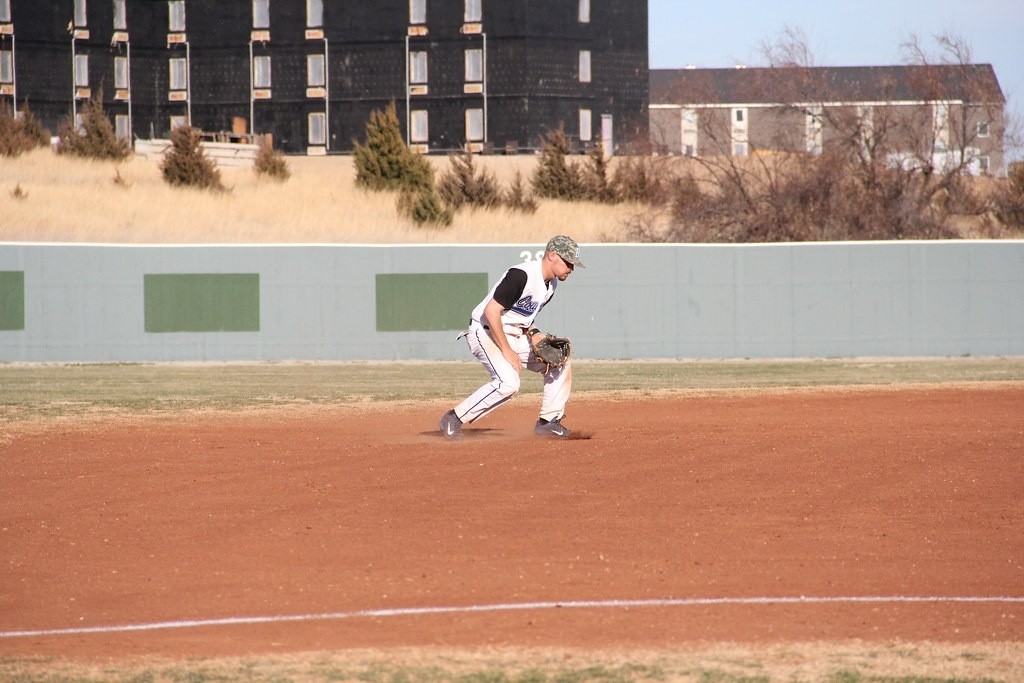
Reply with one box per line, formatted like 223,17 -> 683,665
440,235 -> 586,441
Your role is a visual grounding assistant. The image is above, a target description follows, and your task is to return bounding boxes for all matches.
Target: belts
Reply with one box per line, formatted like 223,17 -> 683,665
469,319 -> 489,329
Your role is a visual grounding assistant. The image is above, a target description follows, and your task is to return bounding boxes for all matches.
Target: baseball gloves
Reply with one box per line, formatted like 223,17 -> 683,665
533,332 -> 572,369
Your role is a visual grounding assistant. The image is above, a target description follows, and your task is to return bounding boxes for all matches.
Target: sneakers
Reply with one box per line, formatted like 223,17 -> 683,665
534,415 -> 574,440
440,410 -> 464,441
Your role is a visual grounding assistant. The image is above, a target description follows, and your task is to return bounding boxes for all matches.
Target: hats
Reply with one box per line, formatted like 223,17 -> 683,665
547,235 -> 584,268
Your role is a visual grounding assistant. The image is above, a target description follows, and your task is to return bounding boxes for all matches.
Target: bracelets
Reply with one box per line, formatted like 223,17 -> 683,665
528,328 -> 540,337
